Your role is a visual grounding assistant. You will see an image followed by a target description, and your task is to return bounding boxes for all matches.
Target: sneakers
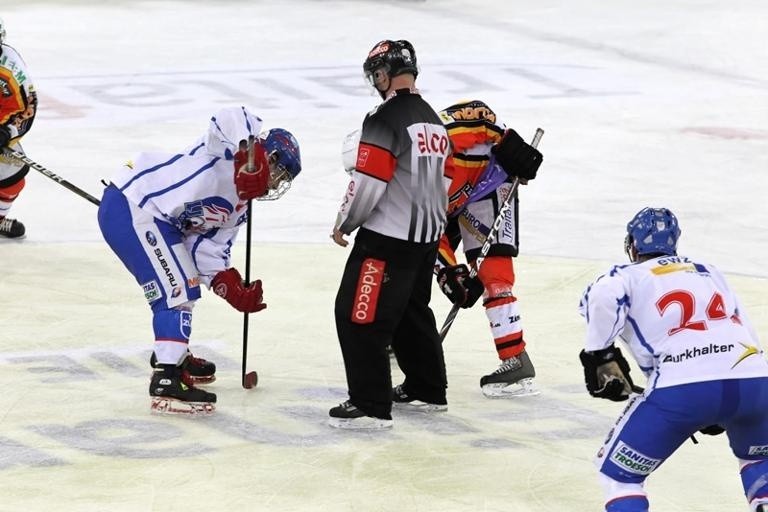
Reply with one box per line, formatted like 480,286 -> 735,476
150,348 -> 215,376
149,363 -> 217,402
329,399 -> 372,419
0,216 -> 24,238
391,383 -> 426,405
480,350 -> 535,388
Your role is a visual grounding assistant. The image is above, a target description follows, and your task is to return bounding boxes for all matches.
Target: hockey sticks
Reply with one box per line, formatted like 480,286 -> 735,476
601,372 -> 698,445
387,129 -> 547,361
242,133 -> 257,387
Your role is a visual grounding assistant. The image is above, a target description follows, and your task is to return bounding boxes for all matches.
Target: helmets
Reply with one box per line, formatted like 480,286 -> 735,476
255,127 -> 301,201
363,40 -> 418,100
341,127 -> 363,179
624,208 -> 680,263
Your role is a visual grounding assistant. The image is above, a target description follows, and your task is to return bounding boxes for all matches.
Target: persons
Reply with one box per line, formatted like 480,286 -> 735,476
97,104 -> 301,404
0,18 -> 38,238
342,98 -> 544,388
579,207 -> 768,512
329,39 -> 452,421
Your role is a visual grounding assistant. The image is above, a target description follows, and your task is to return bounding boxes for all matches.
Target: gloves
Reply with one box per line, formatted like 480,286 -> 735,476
0,123 -> 11,152
233,143 -> 270,200
580,342 -> 634,401
493,129 -> 543,186
437,264 -> 484,309
212,267 -> 267,312
